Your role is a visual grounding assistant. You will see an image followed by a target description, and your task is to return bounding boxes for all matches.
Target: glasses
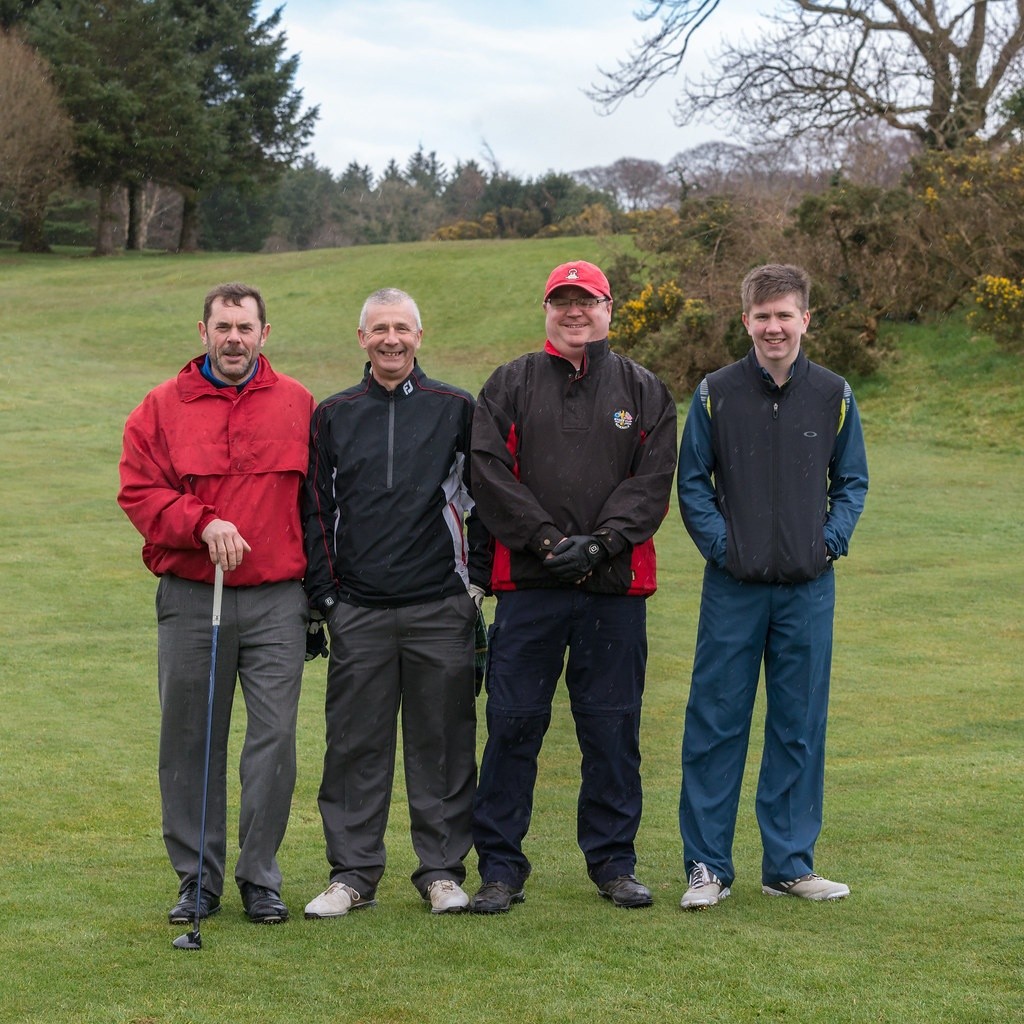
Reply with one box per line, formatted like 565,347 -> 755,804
547,298 -> 608,311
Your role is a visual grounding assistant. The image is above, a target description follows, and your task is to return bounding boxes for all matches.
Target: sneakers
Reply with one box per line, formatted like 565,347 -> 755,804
241,883 -> 290,923
169,883 -> 222,923
428,877 -> 470,915
470,880 -> 525,913
303,883 -> 376,919
679,862 -> 732,908
597,873 -> 653,906
761,874 -> 850,900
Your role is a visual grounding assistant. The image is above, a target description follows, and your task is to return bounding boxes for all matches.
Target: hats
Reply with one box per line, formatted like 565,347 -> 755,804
544,260 -> 613,302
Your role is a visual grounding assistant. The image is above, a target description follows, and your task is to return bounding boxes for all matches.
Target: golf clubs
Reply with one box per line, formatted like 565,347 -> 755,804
170,560 -> 227,951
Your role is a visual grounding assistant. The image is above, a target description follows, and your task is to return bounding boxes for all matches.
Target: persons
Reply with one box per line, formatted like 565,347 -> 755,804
466,259 -> 677,915
677,263 -> 869,909
296,286 -> 494,919
116,282 -> 331,924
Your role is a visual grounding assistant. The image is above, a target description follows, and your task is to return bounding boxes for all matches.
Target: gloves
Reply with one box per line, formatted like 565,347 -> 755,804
467,582 -> 485,623
542,536 -> 610,584
304,608 -> 328,661
